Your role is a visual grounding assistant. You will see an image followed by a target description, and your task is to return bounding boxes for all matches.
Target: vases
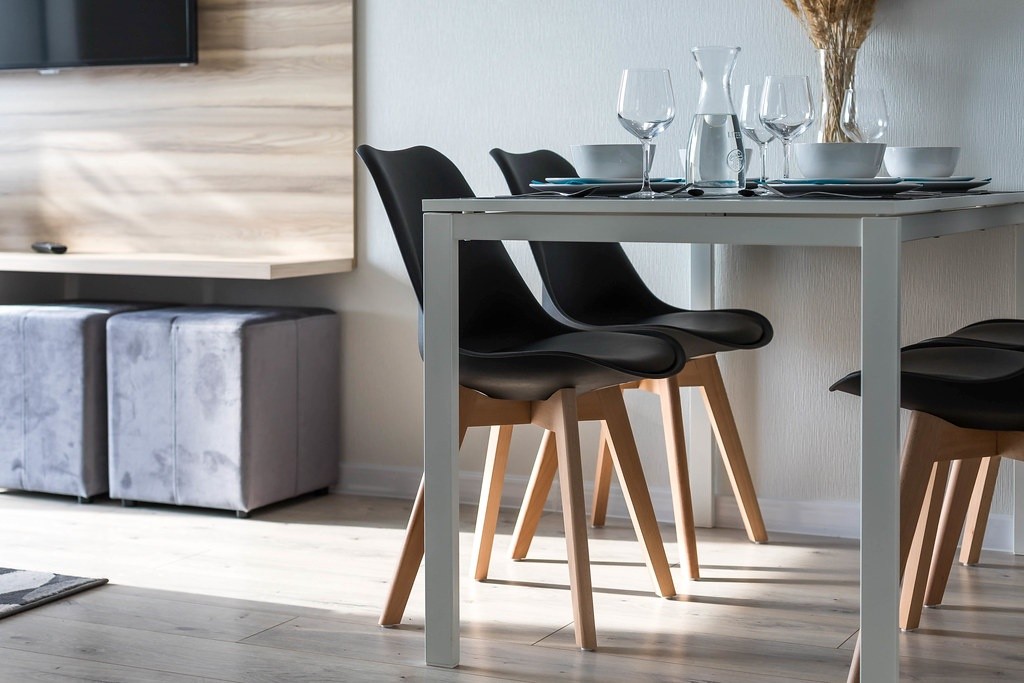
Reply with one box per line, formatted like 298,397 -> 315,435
816,46 -> 861,149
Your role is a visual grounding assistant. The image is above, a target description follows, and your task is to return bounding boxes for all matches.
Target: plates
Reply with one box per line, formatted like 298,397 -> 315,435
899,181 -> 991,193
529,182 -> 684,197
780,177 -> 902,183
874,176 -> 975,181
545,177 -> 665,182
757,184 -> 923,196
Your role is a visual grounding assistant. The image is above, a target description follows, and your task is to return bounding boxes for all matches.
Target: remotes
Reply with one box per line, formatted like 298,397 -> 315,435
31,241 -> 67,254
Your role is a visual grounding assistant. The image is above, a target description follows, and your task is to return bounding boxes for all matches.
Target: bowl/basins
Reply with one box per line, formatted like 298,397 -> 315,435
679,148 -> 753,174
570,144 -> 657,178
794,143 -> 888,178
883,146 -> 962,177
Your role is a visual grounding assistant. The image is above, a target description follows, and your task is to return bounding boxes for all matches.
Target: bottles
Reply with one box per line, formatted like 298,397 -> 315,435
685,45 -> 746,195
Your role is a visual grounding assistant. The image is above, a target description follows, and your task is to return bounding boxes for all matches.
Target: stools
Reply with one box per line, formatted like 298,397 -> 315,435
0,301 -> 177,503
899,320 -> 1024,606
105,307 -> 342,519
828,344 -> 1024,683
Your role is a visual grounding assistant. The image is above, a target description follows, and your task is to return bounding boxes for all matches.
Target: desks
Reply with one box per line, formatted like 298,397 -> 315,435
422,188 -> 1024,683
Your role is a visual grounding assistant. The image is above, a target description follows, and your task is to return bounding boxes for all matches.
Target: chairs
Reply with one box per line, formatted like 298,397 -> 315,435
354,145 -> 686,652
489,148 -> 774,580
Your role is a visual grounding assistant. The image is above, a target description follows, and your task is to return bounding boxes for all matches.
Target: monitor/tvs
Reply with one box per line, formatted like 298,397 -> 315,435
0,0 -> 199,70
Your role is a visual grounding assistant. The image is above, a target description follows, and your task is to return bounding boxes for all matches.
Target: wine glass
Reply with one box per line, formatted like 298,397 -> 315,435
616,69 -> 676,199
739,84 -> 788,184
758,75 -> 815,179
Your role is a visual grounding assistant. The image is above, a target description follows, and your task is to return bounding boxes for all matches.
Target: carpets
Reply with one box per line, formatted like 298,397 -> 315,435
0,567 -> 109,622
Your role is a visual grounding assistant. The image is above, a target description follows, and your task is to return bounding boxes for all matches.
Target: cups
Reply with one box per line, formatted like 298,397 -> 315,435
840,87 -> 888,143
